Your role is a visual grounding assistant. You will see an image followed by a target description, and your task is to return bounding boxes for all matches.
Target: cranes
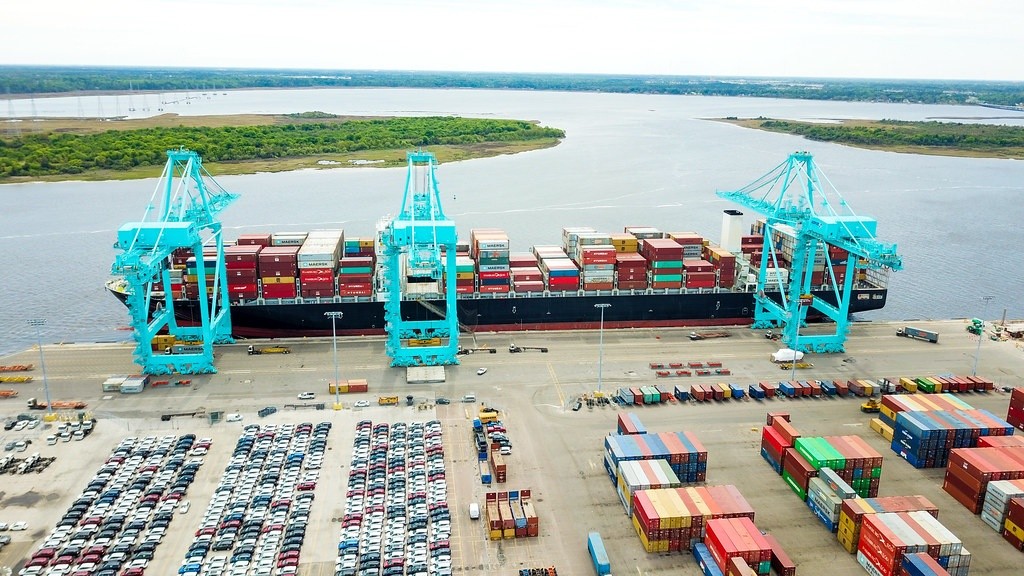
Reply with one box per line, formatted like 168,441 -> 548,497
709,144 -> 909,356
372,145 -> 464,370
107,146 -> 242,387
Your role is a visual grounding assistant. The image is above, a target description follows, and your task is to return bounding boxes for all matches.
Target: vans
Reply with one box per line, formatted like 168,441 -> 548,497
462,395 -> 476,403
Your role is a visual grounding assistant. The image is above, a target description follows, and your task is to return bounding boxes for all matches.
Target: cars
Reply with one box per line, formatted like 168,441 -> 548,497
1,406 -> 514,575
476,367 -> 488,375
354,400 -> 370,407
436,399 -> 450,405
298,392 -> 315,400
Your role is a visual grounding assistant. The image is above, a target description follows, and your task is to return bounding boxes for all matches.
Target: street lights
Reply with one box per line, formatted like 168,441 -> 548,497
594,303 -> 612,398
324,311 -> 345,410
791,299 -> 811,381
970,295 -> 996,376
26,318 -> 53,414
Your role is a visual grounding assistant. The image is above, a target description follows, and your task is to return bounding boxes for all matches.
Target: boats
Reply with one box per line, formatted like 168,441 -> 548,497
106,228 -> 897,331
